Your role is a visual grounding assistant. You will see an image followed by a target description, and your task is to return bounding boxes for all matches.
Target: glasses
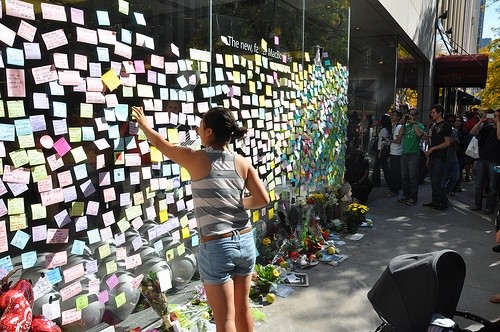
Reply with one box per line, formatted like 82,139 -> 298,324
448,120 -> 455,124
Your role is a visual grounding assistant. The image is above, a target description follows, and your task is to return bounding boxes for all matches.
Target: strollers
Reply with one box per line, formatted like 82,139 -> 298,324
367,249 -> 500,332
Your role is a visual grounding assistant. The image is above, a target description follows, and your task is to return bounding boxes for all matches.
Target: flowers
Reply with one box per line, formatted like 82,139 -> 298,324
255,182 -> 369,287
138,273 -> 171,324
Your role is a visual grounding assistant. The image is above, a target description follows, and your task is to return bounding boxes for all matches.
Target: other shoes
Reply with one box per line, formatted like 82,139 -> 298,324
406,200 -> 416,205
387,192 -> 396,196
447,192 -> 455,196
457,188 -> 462,192
434,203 -> 448,210
423,202 -> 437,206
471,205 -> 481,210
484,208 -> 493,213
465,177 -> 470,182
398,196 -> 407,202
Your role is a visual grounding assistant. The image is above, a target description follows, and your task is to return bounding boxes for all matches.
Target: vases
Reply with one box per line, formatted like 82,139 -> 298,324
260,283 -> 271,292
347,221 -> 359,233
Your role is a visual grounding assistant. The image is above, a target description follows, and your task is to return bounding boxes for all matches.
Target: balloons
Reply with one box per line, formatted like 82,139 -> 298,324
0,206 -> 198,332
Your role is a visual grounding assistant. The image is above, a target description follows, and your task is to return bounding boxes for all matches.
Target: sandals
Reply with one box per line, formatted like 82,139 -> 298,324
490,294 -> 500,302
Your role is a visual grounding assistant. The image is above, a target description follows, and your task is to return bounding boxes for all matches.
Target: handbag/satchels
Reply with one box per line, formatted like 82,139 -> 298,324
381,145 -> 390,160
465,135 -> 479,159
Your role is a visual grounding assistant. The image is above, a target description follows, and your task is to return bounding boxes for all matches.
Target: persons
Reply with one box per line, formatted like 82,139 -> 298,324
347,104 -> 436,206
422,104 -> 452,211
130,105 -> 270,332
445,107 -> 500,253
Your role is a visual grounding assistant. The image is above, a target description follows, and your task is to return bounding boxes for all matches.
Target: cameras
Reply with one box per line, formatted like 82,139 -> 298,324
403,114 -> 411,119
486,114 -> 494,118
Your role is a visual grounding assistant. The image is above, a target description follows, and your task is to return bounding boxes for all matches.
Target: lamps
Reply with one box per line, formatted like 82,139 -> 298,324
438,10 -> 448,19
453,47 -> 458,54
446,27 -> 452,34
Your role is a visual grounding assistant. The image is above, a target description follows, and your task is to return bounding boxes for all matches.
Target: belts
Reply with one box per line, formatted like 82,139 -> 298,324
200,227 -> 252,243
